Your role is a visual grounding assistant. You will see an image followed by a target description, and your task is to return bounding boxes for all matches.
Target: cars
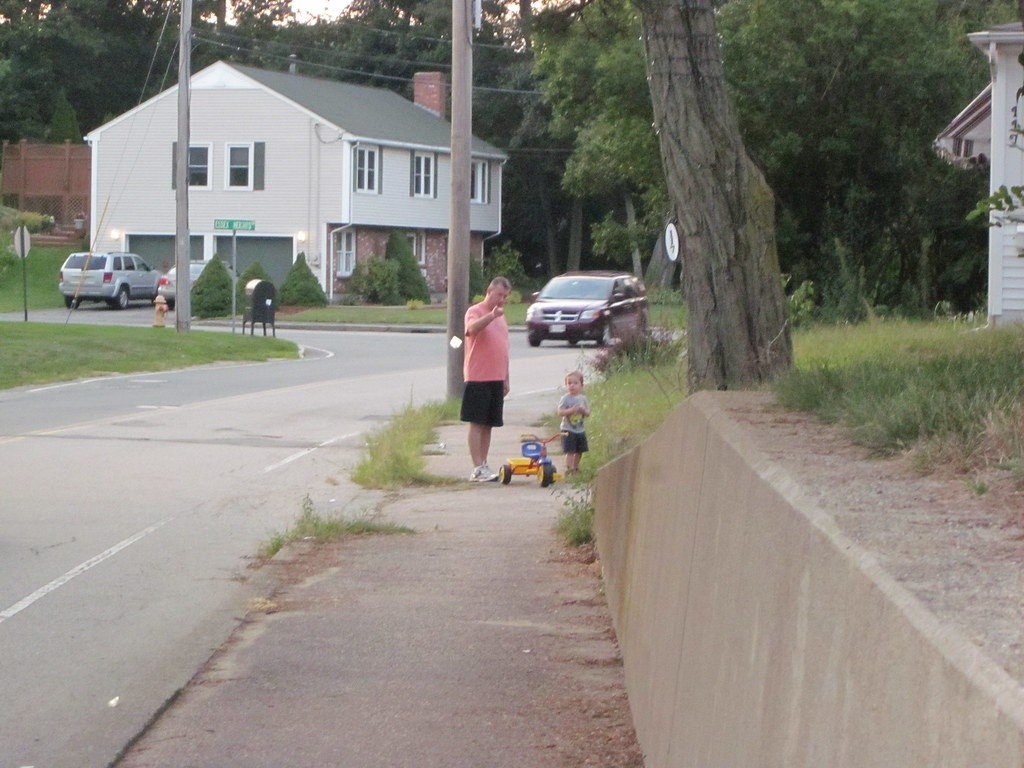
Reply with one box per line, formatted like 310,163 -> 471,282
158,260 -> 236,309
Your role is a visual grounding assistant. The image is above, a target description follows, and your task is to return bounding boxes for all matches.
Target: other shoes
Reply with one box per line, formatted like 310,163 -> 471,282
564,467 -> 581,478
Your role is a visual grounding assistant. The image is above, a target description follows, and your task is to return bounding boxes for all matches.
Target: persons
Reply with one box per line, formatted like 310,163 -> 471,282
555,370 -> 591,476
459,276 -> 510,483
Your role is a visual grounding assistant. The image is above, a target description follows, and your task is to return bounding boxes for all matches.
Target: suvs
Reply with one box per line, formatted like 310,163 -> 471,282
58,251 -> 163,310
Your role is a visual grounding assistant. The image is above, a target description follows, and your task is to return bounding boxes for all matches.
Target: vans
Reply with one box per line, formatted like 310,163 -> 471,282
526,269 -> 648,348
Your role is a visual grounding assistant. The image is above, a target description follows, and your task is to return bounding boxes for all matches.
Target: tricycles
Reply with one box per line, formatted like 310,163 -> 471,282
499,431 -> 569,487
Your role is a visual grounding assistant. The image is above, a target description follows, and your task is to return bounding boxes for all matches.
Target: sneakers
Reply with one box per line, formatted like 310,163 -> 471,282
469,460 -> 499,481
